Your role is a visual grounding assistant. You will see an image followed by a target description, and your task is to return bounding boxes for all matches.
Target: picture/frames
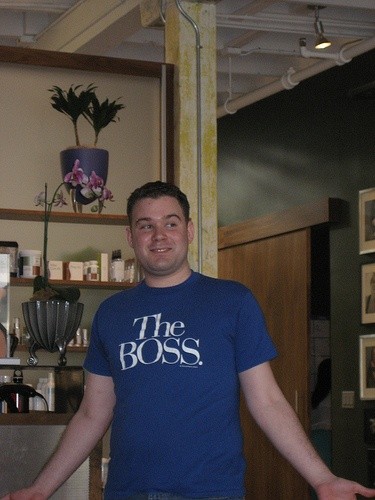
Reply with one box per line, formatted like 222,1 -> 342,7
360,334 -> 375,402
357,186 -> 375,256
361,263 -> 375,325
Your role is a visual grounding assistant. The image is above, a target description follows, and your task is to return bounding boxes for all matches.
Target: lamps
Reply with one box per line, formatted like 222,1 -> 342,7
308,4 -> 332,51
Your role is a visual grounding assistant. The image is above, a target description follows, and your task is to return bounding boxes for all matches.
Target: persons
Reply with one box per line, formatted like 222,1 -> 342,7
0,180 -> 375,500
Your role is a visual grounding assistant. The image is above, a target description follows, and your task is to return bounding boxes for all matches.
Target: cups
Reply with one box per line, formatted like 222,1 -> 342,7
75,328 -> 88,347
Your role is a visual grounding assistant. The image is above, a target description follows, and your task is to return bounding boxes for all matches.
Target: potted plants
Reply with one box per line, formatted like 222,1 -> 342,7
45,81 -> 128,205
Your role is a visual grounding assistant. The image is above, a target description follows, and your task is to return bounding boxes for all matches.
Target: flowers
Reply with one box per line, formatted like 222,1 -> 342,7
29,157 -> 120,300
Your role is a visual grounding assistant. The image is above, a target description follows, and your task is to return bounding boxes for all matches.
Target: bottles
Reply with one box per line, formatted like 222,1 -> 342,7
124,264 -> 136,283
111,249 -> 124,282
13,317 -> 21,345
83,260 -> 100,281
17,250 -> 41,279
0,241 -> 19,278
0,372 -> 56,412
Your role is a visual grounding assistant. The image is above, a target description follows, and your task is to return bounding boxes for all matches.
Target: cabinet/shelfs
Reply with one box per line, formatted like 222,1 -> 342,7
0,208 -> 139,352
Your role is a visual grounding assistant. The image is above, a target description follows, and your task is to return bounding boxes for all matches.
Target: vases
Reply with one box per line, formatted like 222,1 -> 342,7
22,300 -> 85,368
